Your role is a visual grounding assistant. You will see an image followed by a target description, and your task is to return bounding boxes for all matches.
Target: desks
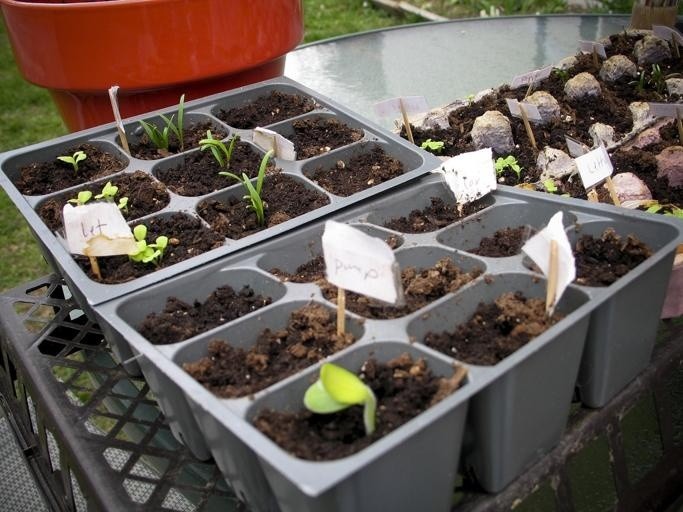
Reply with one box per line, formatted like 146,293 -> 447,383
254,14 -> 680,141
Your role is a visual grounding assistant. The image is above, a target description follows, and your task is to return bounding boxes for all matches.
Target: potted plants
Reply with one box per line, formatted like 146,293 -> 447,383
0,94 -> 339,307
238,341 -> 470,512
